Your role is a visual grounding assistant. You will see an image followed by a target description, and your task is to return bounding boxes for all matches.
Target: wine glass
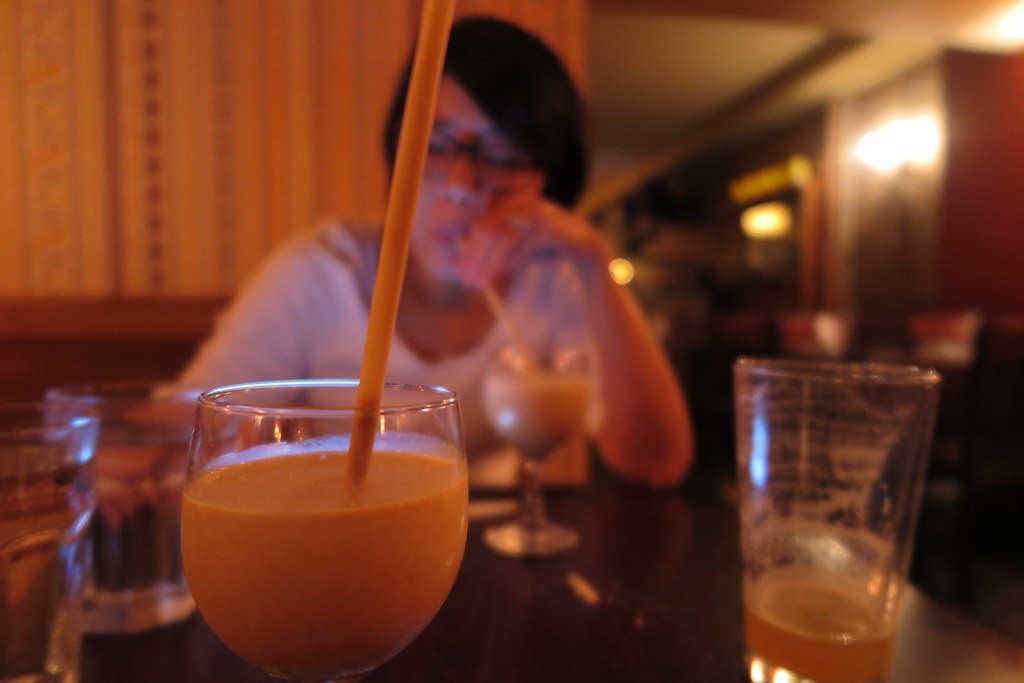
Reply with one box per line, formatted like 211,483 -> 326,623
485,257 -> 599,554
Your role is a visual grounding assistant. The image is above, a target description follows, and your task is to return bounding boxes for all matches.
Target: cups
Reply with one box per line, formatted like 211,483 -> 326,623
733,356 -> 942,683
183,379 -> 470,683
0,401 -> 99,683
44,383 -> 206,632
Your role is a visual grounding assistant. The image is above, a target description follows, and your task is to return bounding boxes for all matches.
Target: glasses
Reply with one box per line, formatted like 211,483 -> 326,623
424,121 -> 536,193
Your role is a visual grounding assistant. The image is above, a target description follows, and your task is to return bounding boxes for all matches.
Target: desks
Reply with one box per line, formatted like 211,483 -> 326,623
0,451 -> 1024,683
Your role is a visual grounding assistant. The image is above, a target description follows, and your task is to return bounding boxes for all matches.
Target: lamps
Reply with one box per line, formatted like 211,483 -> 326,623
727,156 -> 814,238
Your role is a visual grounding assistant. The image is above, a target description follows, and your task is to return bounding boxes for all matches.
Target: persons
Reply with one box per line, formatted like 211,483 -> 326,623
180,12 -> 692,486
